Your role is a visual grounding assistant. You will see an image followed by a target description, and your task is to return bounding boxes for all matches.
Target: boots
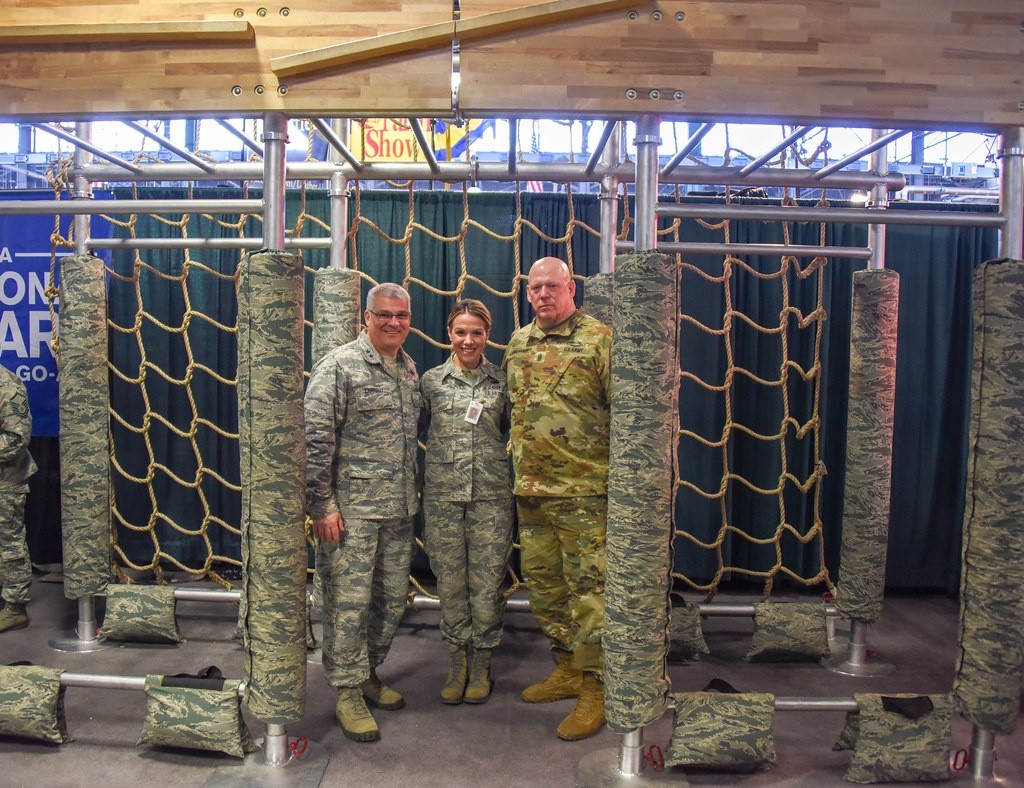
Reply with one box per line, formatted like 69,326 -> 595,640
522,652 -> 581,703
556,671 -> 607,741
334,685 -> 380,742
361,667 -> 404,710
440,647 -> 468,704
465,647 -> 491,704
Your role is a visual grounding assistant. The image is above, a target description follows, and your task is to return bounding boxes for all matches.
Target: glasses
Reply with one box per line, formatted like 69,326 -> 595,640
370,310 -> 411,320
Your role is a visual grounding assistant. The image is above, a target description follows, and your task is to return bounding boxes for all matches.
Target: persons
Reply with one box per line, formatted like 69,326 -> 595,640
0,363 -> 37,634
500,256 -> 607,741
419,299 -> 516,704
305,283 -> 425,742
469,409 -> 477,418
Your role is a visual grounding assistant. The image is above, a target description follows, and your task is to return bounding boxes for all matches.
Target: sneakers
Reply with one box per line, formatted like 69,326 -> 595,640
0,602 -> 28,632
